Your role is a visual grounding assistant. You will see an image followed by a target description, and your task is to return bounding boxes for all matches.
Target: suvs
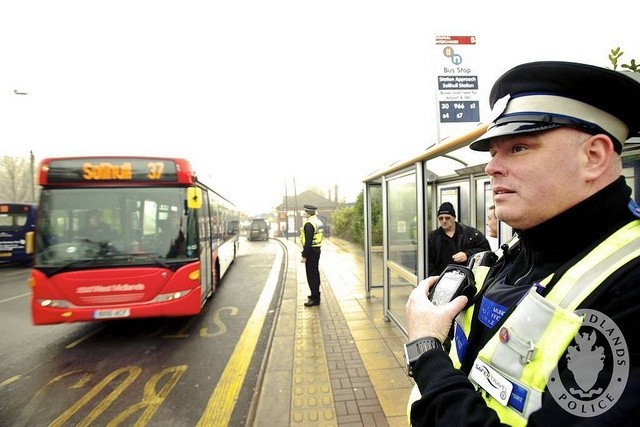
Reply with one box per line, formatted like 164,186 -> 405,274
247,218 -> 268,240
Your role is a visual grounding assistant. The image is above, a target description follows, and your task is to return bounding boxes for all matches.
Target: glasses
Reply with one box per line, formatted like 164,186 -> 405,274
439,216 -> 450,220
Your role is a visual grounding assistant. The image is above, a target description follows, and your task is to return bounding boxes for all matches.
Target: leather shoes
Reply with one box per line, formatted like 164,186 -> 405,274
304,300 -> 319,306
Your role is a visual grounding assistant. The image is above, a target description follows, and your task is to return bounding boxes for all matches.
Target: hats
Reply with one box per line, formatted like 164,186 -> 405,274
437,202 -> 455,217
470,61 -> 640,155
304,205 -> 317,211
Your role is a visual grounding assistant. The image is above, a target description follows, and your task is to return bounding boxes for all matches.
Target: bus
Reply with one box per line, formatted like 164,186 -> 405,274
0,201 -> 38,265
27,156 -> 240,324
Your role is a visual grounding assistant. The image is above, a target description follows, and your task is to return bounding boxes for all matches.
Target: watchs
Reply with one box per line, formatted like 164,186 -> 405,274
402,336 -> 450,378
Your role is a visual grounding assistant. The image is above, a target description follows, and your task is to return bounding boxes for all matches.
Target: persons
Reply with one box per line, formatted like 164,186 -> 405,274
486,204 -> 497,239
402,60 -> 639,426
75,209 -> 115,246
426,201 -> 492,354
298,204 -> 325,307
